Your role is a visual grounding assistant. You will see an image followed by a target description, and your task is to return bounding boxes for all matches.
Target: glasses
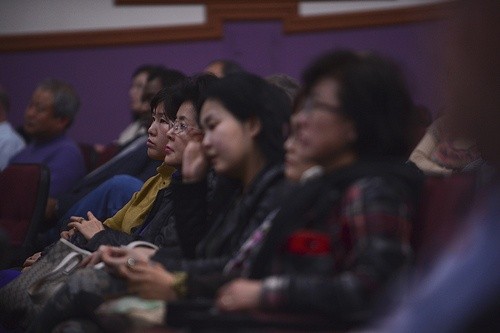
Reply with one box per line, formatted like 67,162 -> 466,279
169,120 -> 195,134
304,97 -> 342,118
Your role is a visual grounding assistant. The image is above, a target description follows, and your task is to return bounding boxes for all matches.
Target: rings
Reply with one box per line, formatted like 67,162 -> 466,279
78,217 -> 84,224
29,256 -> 32,260
126,254 -> 136,270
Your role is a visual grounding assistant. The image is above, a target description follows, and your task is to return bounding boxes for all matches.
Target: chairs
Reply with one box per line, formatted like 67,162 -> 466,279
0,162 -> 51,272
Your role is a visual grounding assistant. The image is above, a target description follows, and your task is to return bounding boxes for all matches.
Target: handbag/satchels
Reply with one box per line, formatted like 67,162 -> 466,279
0,237 -> 159,333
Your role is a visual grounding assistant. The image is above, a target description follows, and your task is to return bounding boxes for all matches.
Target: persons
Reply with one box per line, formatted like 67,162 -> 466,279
0,46 -> 435,333
